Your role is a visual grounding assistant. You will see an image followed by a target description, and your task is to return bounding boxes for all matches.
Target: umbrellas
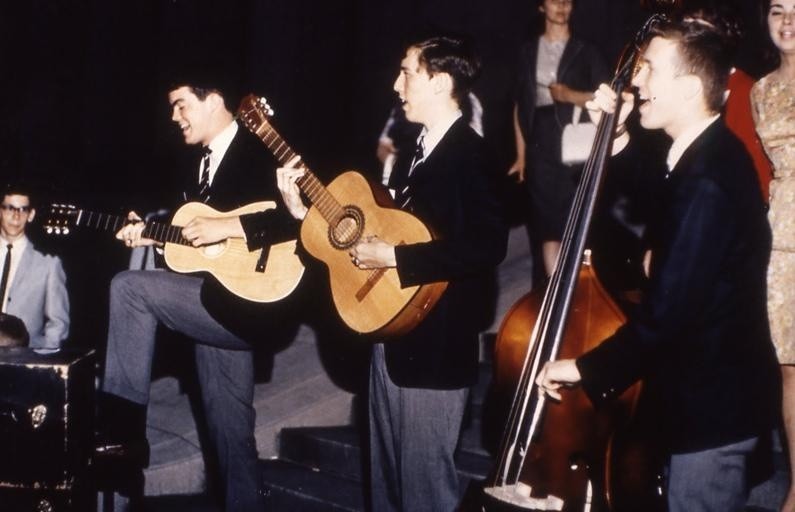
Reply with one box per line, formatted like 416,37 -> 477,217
91,440 -> 149,467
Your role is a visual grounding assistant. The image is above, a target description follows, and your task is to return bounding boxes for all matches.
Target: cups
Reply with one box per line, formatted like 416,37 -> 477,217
1,205 -> 30,212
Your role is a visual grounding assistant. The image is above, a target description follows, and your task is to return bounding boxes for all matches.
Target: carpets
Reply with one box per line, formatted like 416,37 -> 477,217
197,145 -> 212,205
0,245 -> 12,313
400,135 -> 425,212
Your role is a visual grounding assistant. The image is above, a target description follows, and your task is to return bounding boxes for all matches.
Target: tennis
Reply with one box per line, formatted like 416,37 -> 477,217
487,12 -> 668,512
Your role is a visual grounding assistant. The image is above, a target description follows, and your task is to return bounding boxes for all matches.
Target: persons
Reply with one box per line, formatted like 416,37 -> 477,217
0,185 -> 74,363
375,87 -> 486,200
96,68 -> 308,511
504,1 -> 611,285
273,31 -> 512,511
745,0 -> 795,512
1,308 -> 38,353
528,6 -> 781,512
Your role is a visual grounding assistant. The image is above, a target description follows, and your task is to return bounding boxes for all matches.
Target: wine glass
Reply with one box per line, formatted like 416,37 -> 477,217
127,239 -> 132,244
351,256 -> 360,266
